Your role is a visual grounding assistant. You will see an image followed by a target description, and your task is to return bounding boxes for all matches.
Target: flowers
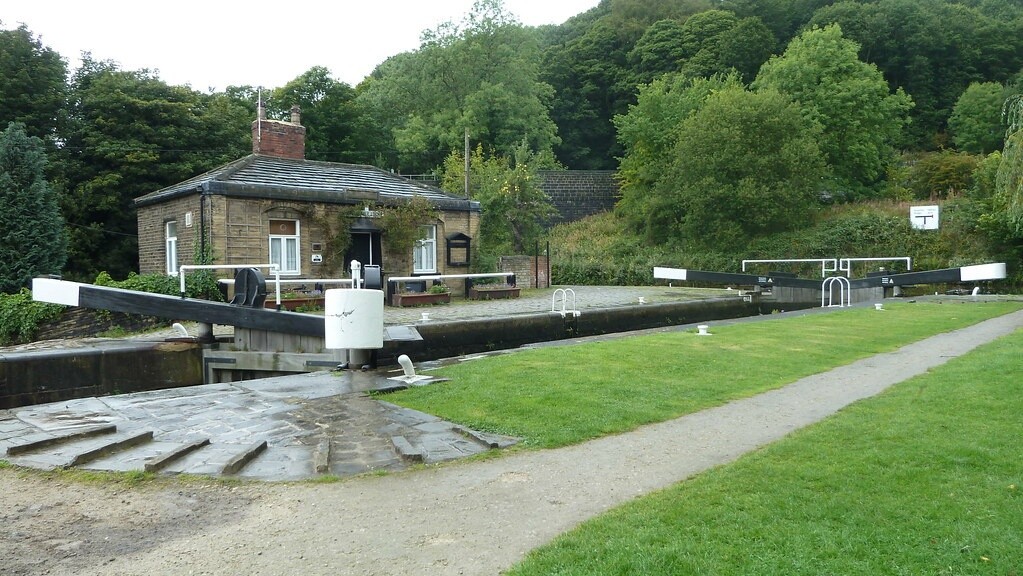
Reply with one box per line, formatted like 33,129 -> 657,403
398,284 -> 450,294
267,290 -> 321,299
474,284 -> 512,289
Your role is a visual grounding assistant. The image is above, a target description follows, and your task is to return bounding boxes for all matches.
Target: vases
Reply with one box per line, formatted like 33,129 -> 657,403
470,288 -> 521,300
391,294 -> 452,307
266,298 -> 325,311
